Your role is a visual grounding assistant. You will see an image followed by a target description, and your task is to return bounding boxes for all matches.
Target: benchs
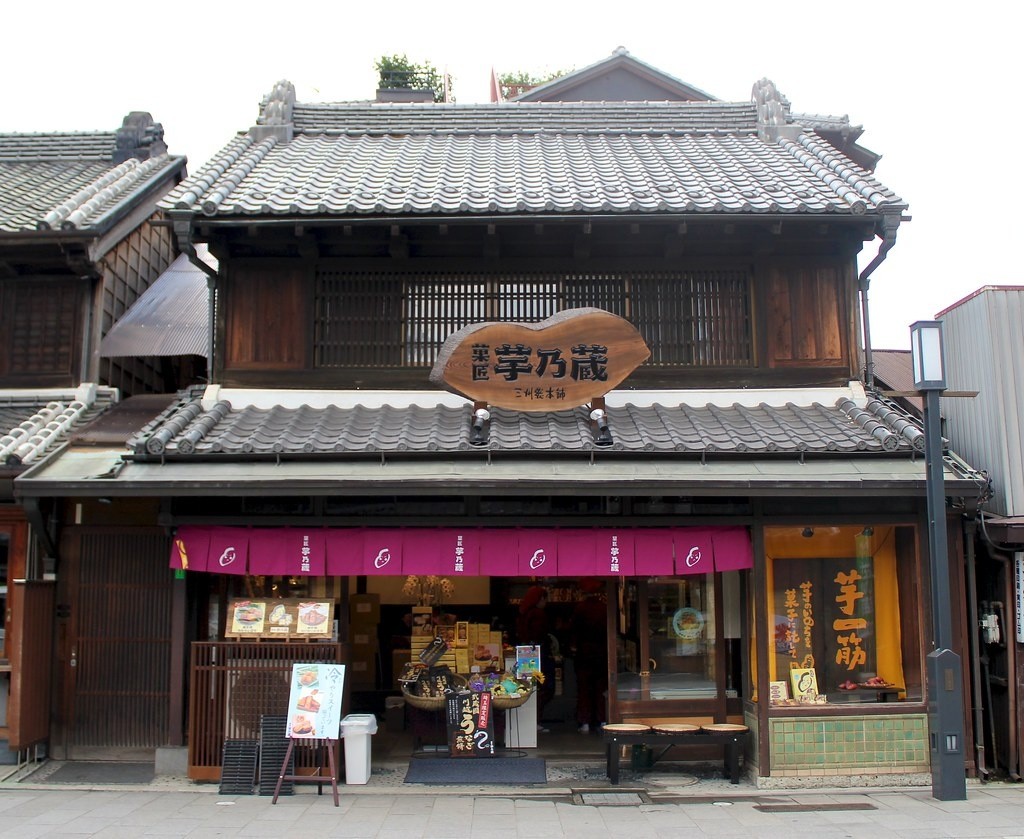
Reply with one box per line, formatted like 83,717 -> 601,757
600,723 -> 751,786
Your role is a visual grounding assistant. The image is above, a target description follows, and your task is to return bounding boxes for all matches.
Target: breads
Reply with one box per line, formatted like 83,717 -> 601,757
302,610 -> 325,624
296,695 -> 320,712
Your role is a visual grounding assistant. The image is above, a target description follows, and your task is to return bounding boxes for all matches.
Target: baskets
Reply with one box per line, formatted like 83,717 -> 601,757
467,672 -> 534,708
401,672 -> 467,709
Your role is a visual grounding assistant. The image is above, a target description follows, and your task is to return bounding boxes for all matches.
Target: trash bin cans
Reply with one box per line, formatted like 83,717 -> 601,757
340,714 -> 376,784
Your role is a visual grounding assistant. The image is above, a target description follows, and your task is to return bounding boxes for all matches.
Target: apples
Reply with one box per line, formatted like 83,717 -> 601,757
868,676 -> 883,684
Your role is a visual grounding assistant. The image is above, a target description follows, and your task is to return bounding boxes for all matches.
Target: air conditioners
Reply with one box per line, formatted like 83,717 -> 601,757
224,658 -> 335,752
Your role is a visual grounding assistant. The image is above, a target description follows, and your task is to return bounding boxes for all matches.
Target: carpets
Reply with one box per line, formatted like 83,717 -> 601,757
403,758 -> 548,786
45,761 -> 155,785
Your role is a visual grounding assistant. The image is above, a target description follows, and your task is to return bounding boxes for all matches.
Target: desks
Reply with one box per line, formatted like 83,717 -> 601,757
837,686 -> 905,703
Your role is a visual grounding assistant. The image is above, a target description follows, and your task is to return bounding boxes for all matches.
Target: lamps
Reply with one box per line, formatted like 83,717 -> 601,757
862,527 -> 874,536
590,408 -> 609,440
801,527 -> 813,537
472,408 -> 491,440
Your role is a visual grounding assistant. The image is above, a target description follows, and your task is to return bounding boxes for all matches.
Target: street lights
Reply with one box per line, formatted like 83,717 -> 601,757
909,315 -> 968,803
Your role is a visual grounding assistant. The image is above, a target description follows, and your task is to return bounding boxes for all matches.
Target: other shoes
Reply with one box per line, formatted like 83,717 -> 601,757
537,725 -> 549,733
577,724 -> 589,735
597,723 -> 606,733
